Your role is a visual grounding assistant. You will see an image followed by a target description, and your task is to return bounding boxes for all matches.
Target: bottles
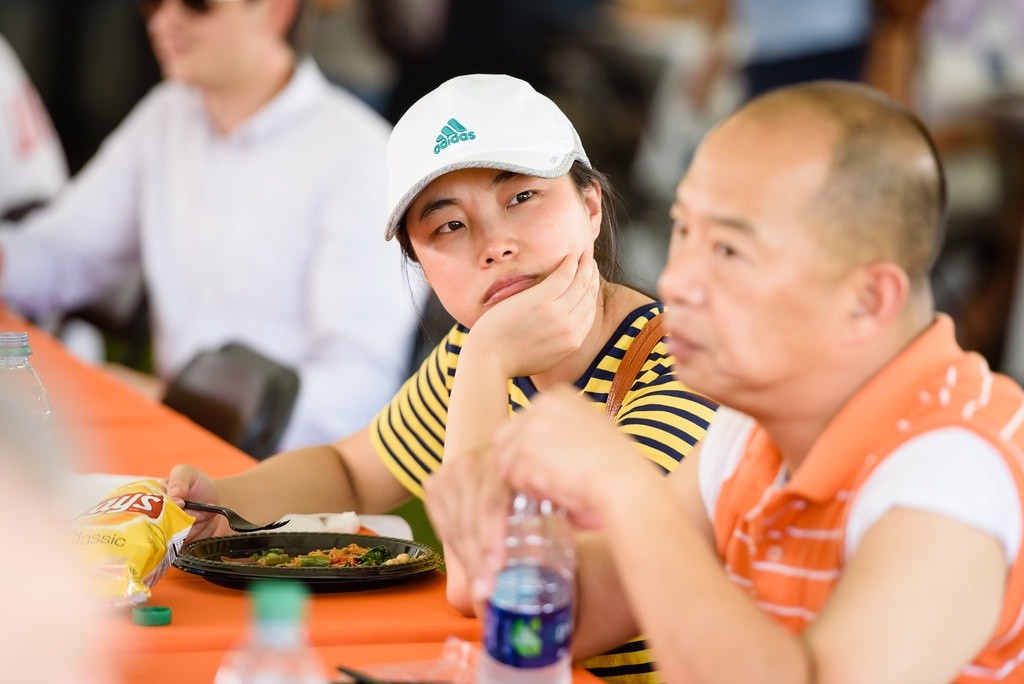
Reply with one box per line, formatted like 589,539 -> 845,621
214,582 -> 328,684
479,492 -> 576,684
0,333 -> 55,459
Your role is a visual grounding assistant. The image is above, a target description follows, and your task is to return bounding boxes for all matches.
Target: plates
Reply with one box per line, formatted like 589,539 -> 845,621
173,532 -> 444,592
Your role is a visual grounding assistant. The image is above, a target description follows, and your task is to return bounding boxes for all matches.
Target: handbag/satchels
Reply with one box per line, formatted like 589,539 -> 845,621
161,344 -> 301,462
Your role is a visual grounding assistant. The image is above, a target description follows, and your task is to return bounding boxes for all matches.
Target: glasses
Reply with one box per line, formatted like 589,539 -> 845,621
140,0 -> 212,16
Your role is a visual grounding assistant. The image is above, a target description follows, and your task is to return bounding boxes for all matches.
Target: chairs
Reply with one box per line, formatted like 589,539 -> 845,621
165,339 -> 303,466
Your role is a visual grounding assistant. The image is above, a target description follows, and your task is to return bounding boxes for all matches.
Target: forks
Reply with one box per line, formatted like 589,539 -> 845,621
182,499 -> 291,532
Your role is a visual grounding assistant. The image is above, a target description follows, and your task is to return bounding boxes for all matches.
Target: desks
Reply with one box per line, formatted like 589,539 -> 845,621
0,297 -> 602,684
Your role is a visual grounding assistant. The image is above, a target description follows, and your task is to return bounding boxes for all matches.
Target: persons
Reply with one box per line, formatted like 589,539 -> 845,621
424,82 -> 1024,684
0,0 -> 433,459
289,0 -> 1024,390
160,74 -> 720,684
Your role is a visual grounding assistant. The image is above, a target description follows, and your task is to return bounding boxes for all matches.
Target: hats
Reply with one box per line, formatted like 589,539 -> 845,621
384,73 -> 594,242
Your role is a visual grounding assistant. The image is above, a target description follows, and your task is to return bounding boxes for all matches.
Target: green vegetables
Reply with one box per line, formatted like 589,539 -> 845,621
253,545 -> 395,569
